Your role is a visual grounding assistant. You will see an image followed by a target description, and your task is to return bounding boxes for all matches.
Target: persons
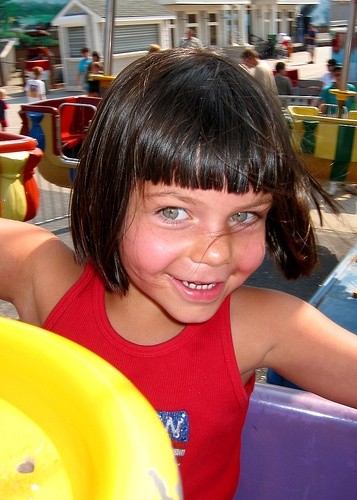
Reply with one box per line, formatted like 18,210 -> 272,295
0,24 -> 355,132
0,46 -> 357,500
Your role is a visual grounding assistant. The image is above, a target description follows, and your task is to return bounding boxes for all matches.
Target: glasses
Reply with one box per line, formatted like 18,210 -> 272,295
327,64 -> 333,66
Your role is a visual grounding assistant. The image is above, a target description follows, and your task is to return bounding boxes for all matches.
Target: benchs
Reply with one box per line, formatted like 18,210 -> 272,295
0,93 -> 103,221
282,101 -> 357,164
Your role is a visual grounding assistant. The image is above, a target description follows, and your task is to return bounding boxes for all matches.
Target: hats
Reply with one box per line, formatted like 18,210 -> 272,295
334,63 -> 343,69
